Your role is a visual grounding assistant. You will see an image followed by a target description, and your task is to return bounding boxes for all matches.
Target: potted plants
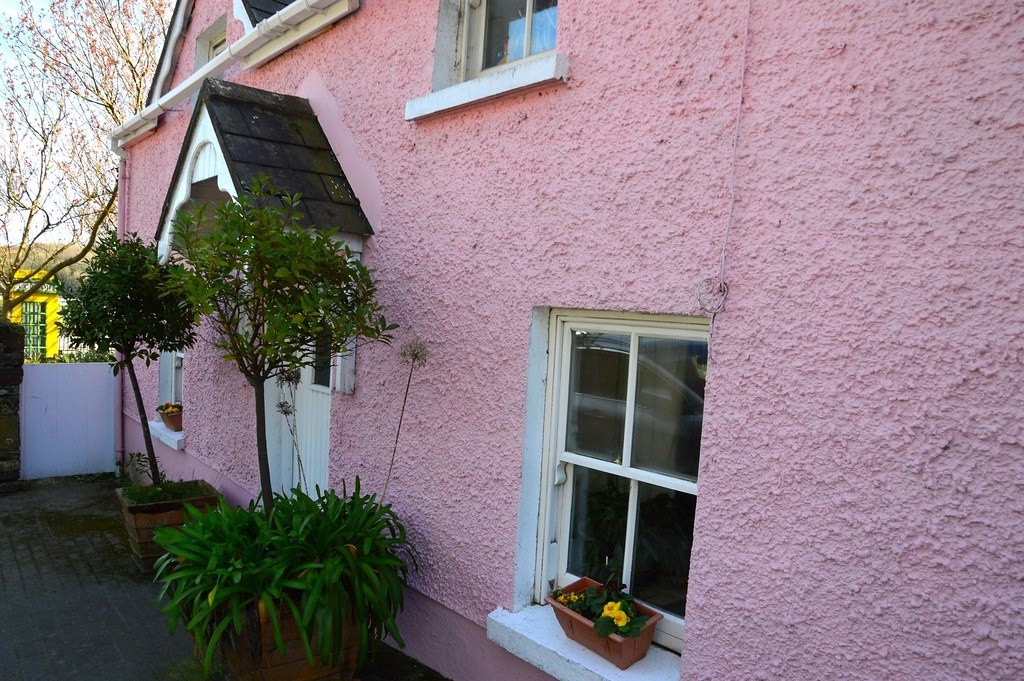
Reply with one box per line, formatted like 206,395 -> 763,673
53,229 -> 222,574
154,168 -> 417,681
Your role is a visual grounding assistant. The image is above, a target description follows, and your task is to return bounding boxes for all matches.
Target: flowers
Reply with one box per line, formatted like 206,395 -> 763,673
553,571 -> 649,639
156,403 -> 182,413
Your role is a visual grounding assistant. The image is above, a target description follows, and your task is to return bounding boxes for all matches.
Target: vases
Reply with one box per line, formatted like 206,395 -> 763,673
543,577 -> 664,671
159,412 -> 182,432
